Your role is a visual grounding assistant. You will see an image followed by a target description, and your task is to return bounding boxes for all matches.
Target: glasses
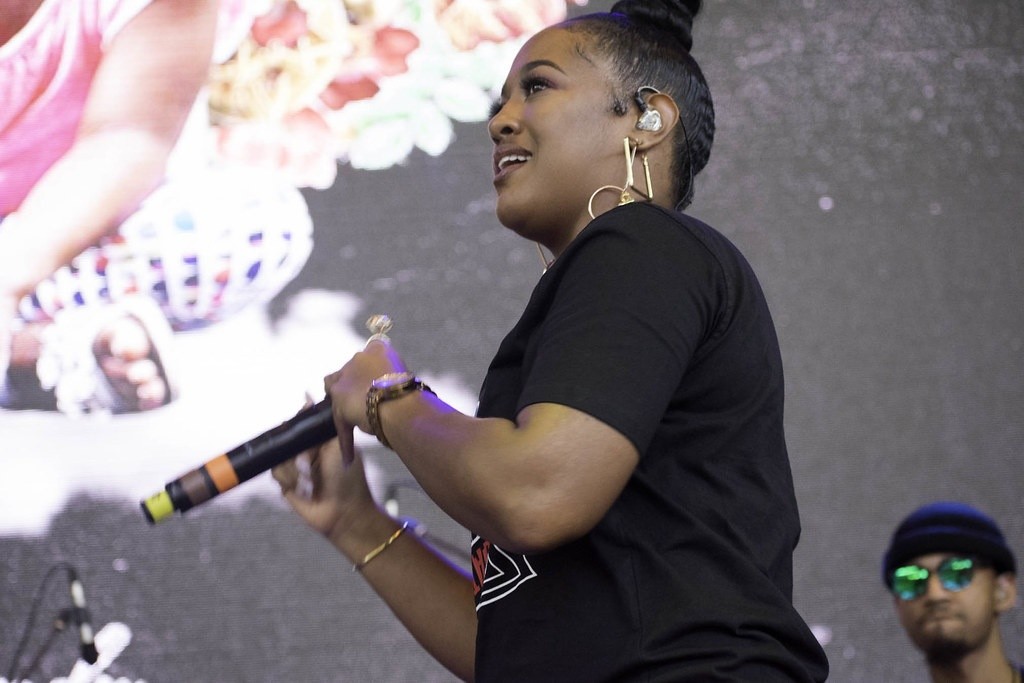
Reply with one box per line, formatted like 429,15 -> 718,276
889,556 -> 996,601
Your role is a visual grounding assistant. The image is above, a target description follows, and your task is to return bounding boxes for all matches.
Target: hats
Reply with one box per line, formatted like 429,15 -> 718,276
881,501 -> 1018,593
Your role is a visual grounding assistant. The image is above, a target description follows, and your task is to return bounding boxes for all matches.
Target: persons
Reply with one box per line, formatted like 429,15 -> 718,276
882,502 -> 1024,683
0,0 -> 309,413
268,1 -> 830,683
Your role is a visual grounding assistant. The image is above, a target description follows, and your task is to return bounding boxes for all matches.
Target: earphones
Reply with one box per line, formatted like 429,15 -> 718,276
636,107 -> 663,131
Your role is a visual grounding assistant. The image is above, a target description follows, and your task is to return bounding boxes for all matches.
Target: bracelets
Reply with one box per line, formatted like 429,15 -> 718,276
351,521 -> 410,571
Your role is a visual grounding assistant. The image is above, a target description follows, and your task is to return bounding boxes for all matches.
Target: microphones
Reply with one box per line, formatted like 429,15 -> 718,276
66,565 -> 98,666
139,396 -> 340,525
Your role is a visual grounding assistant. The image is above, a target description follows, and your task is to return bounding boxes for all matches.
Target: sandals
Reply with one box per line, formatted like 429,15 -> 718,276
2,295 -> 179,414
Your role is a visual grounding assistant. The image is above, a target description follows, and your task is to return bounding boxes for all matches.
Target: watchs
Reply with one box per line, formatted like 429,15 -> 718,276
365,370 -> 437,448
